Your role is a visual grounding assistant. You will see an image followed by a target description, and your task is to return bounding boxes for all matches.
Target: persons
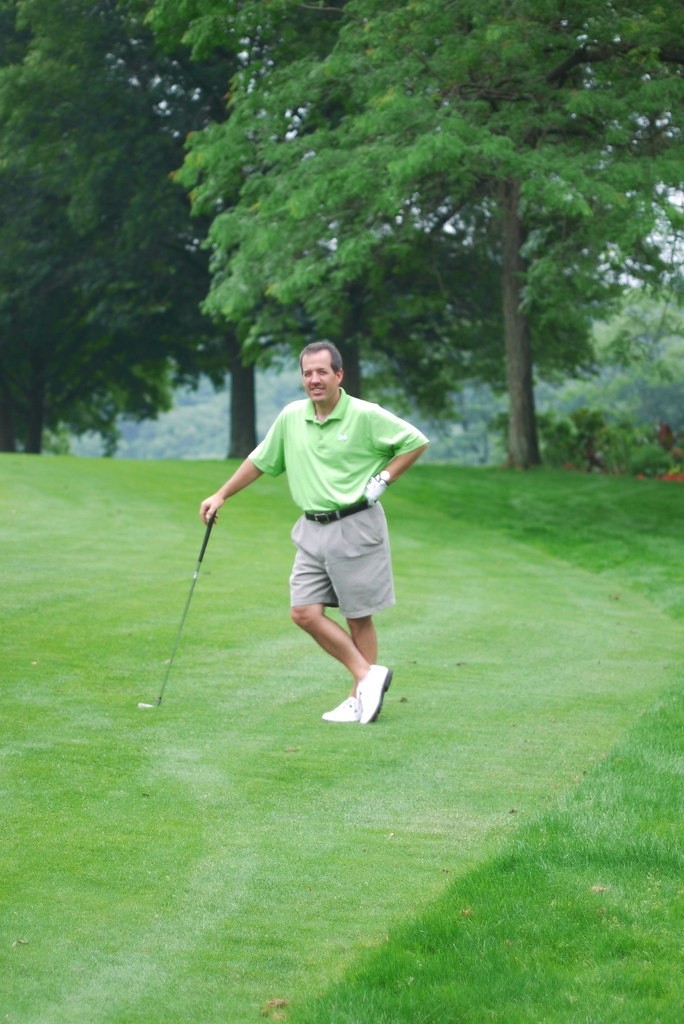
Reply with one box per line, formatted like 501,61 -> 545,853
199,341 -> 430,723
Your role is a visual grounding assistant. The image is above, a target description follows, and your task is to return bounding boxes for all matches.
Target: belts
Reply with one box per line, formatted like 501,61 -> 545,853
304,500 -> 370,525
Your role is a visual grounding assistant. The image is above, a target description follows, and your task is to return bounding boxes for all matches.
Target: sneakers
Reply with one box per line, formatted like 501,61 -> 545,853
321,697 -> 359,724
356,664 -> 393,724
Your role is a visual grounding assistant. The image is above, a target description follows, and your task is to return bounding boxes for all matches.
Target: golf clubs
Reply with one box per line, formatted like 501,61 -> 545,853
137,511 -> 216,710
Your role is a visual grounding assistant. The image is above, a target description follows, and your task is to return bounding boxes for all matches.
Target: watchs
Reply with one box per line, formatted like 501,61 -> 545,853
376,470 -> 391,485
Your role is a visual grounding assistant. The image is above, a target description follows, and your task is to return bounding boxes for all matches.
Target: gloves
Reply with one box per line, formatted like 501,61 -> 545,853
365,478 -> 388,507
